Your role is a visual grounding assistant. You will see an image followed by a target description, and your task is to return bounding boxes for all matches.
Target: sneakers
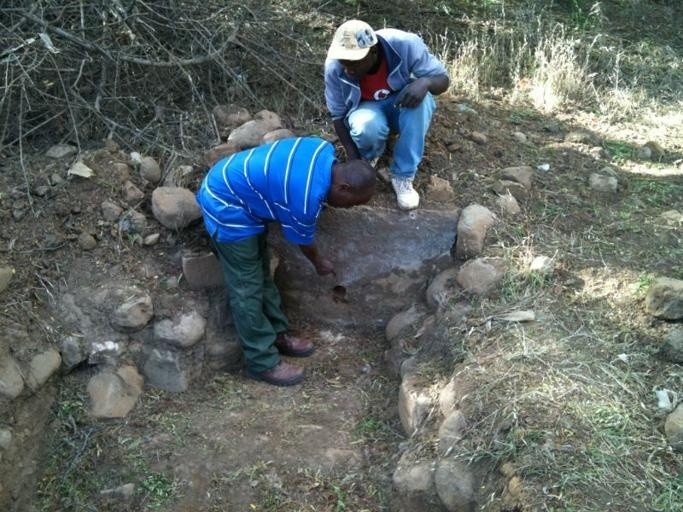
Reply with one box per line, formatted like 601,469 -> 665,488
274,331 -> 312,358
391,175 -> 420,211
246,362 -> 302,385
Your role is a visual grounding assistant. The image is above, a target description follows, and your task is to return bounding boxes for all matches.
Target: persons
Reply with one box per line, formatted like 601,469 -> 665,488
324,19 -> 450,210
197,136 -> 377,387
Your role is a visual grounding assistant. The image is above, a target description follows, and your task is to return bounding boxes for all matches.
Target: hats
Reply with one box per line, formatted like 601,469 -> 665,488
328,20 -> 377,61
